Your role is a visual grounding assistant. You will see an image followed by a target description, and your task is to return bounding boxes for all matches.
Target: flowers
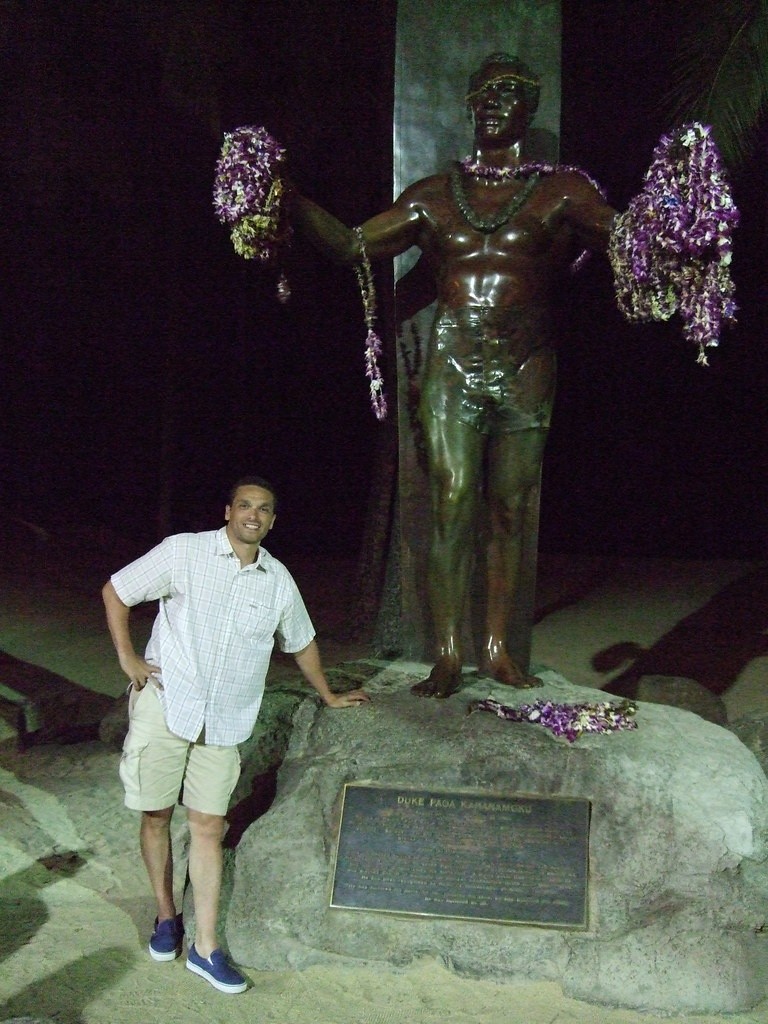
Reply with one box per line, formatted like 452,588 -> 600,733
468,693 -> 639,744
459,161 -> 614,273
352,224 -> 390,423
607,119 -> 743,367
210,122 -> 290,261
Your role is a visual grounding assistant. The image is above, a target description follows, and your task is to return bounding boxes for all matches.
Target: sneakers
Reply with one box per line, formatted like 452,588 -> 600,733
149,914 -> 183,962
186,942 -> 247,993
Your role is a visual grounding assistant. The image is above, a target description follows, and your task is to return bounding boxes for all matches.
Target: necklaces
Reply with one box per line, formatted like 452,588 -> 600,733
443,158 -> 539,232
460,153 -> 608,276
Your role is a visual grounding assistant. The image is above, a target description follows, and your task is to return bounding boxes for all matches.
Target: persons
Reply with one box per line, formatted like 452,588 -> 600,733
212,52 -> 738,699
102,477 -> 371,992
